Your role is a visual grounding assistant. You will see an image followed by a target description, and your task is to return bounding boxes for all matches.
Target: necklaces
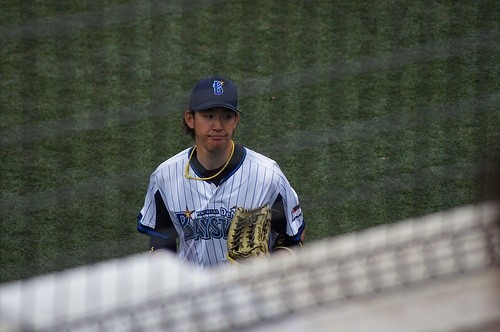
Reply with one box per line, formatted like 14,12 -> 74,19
184,139 -> 235,180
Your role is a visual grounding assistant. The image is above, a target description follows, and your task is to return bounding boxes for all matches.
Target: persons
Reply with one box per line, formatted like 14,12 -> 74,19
137,76 -> 306,269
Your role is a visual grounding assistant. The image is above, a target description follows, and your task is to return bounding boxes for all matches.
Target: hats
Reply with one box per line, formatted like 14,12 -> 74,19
189,77 -> 241,116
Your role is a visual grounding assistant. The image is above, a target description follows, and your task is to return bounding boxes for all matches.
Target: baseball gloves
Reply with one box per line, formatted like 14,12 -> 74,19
224,201 -> 273,267
